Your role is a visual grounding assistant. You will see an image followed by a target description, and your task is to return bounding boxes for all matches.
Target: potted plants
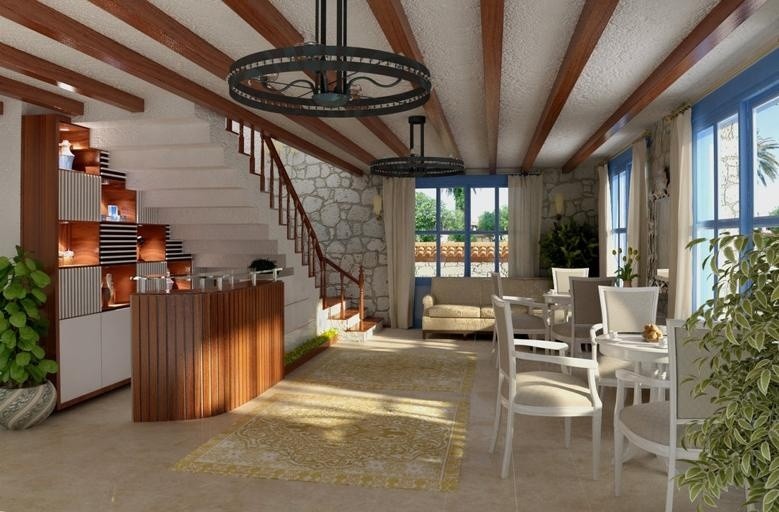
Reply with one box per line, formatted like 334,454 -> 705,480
0,246 -> 58,430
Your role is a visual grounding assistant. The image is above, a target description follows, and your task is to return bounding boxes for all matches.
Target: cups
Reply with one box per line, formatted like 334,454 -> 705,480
59,247 -> 74,258
659,337 -> 667,346
59,259 -> 74,263
100,215 -> 127,222
550,288 -> 556,294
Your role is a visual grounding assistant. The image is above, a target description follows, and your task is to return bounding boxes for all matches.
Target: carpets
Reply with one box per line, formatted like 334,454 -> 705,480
168,343 -> 479,492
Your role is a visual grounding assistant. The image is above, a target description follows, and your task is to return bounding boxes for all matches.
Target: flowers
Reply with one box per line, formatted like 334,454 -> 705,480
611,246 -> 642,282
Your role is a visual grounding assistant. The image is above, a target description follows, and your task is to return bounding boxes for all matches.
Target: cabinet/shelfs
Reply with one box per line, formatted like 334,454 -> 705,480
22,110 -> 198,411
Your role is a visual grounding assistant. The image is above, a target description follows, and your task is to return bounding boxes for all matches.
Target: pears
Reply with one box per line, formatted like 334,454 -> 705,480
643,323 -> 662,341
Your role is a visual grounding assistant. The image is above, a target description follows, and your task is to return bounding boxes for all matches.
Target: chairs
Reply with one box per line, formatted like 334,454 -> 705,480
590,286 -> 670,402
614,319 -> 756,512
490,295 -> 602,481
495,268 -> 617,376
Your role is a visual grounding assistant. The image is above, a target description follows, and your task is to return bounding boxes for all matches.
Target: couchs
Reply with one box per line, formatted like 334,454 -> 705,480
419,274 -> 553,339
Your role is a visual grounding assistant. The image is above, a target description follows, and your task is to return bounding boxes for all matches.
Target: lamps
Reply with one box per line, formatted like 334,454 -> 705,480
556,192 -> 565,221
226,0 -> 433,120
368,117 -> 468,182
371,196 -> 383,219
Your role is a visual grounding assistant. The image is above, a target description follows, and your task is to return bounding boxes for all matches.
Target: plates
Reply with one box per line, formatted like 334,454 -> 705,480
655,344 -> 668,350
